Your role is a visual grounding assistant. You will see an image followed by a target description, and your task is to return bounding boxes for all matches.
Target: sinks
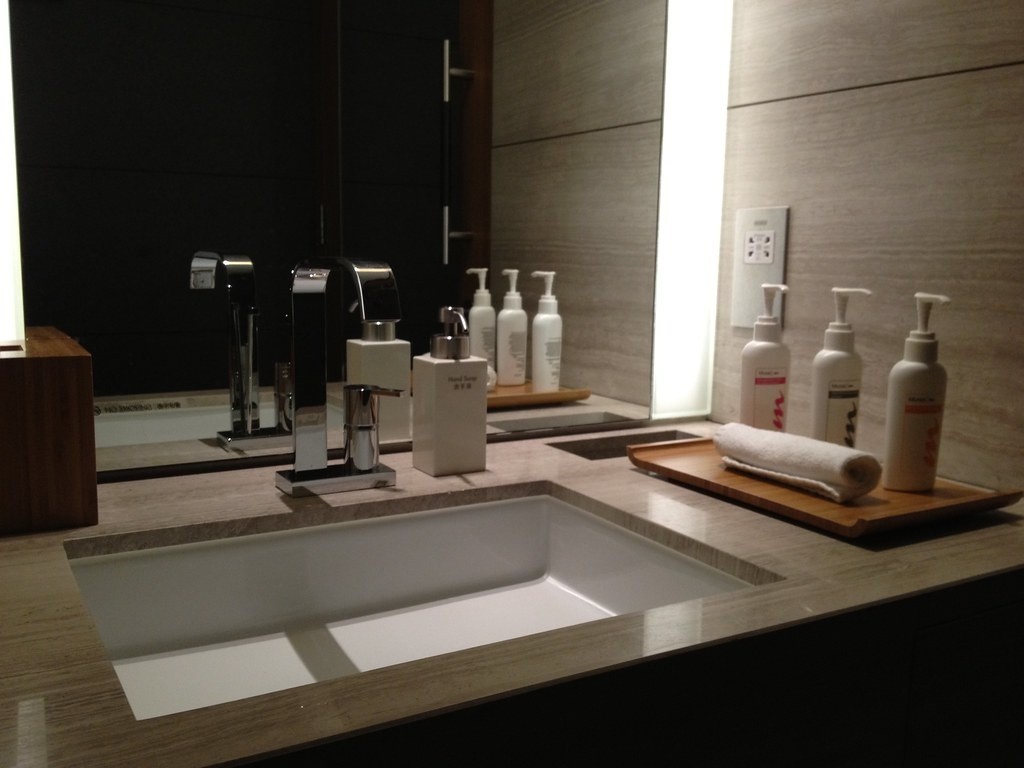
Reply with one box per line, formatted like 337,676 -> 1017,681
62,478 -> 790,720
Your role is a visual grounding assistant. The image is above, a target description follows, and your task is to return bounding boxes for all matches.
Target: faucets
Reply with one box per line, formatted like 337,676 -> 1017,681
188,247 -> 263,435
338,259 -> 406,490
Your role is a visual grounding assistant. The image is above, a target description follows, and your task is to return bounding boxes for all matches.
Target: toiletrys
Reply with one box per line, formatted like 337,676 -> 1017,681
411,305 -> 490,479
465,266 -> 564,395
345,292 -> 412,443
738,282 -> 952,494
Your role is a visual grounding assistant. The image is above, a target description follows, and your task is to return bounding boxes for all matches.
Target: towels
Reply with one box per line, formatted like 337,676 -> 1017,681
712,422 -> 883,503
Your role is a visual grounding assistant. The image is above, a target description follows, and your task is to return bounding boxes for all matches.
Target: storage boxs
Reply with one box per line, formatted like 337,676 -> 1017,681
0,326 -> 100,537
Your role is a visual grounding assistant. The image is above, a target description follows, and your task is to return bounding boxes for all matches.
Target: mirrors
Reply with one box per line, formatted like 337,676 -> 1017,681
10,1 -> 672,471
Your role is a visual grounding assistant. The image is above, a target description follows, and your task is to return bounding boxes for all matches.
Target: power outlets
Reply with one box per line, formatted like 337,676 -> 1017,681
743,228 -> 772,265
